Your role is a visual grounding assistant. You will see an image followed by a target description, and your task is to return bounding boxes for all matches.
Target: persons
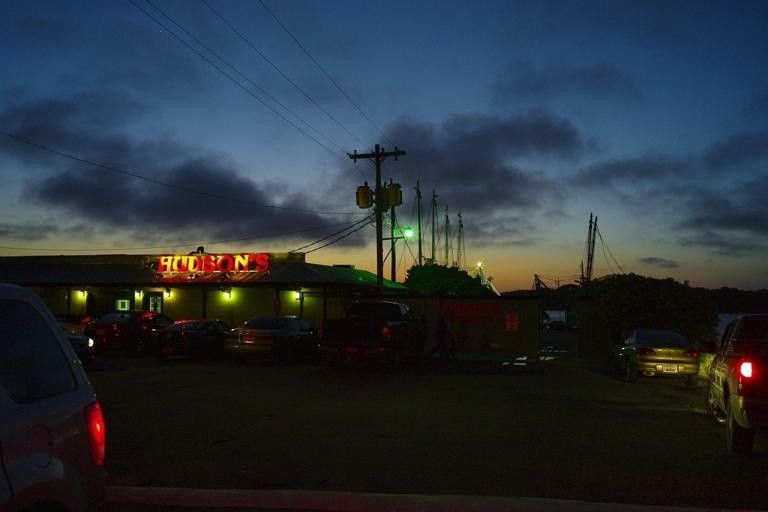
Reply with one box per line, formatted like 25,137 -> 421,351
319,320 -> 329,339
435,310 -> 456,361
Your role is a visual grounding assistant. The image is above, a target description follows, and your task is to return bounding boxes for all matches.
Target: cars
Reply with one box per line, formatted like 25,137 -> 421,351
607,328 -> 700,385
0,283 -> 108,512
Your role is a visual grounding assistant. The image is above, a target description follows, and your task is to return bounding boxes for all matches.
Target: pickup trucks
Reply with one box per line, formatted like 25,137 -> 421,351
702,314 -> 768,456
